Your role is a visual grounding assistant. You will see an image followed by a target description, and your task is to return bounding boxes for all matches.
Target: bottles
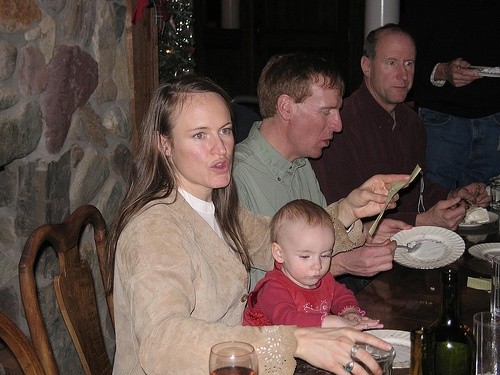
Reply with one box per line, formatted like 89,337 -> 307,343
421,269 -> 476,375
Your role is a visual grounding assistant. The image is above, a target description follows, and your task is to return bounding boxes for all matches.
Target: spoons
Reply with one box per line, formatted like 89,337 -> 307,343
365,243 -> 422,253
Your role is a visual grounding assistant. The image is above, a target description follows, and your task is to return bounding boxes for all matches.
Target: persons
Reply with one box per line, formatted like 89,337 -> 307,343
409,0 -> 500,213
230,51 -> 413,292
105,74 -> 413,375
307,22 -> 492,232
240,198 -> 385,331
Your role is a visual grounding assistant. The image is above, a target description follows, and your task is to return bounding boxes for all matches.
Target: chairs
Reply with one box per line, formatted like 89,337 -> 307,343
18,205 -> 116,375
0,314 -> 44,375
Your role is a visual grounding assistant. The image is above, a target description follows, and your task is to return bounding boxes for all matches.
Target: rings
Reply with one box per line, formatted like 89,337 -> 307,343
350,344 -> 360,358
345,360 -> 355,373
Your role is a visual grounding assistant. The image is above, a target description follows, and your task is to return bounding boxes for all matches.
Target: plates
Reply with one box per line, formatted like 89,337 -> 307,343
467,243 -> 500,263
468,66 -> 500,77
459,209 -> 499,226
390,225 -> 466,269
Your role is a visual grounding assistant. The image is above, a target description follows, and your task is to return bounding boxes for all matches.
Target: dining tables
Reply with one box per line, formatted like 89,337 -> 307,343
291,205 -> 500,374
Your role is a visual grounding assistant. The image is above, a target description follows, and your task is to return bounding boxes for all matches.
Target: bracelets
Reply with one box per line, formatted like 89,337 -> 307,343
344,223 -> 355,234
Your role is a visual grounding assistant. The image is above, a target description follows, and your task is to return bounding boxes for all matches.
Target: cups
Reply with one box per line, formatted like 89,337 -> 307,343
490,255 -> 500,317
473,312 -> 500,375
209,341 -> 259,375
357,342 -> 397,375
490,177 -> 500,211
